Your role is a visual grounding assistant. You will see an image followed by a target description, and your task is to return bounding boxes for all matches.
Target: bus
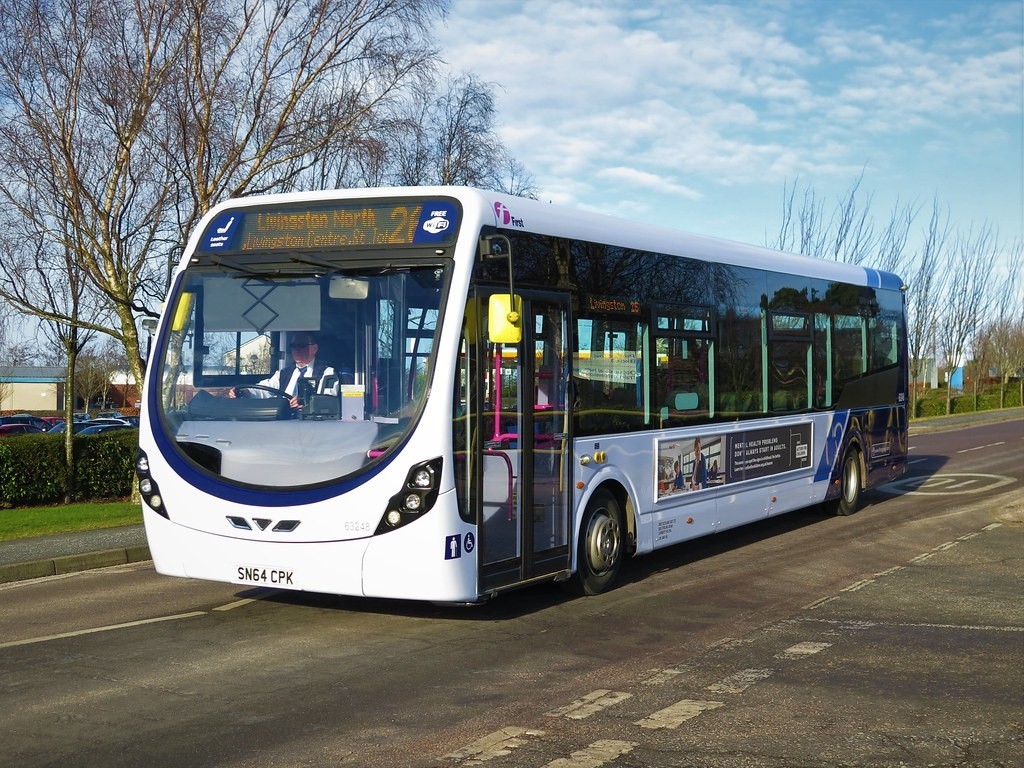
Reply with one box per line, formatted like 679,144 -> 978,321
136,187 -> 910,605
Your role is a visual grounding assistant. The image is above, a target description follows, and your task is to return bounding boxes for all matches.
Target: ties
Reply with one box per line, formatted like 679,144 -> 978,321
292,366 -> 307,396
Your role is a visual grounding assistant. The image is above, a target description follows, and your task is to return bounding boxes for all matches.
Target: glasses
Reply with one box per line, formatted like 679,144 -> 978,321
290,343 -> 314,348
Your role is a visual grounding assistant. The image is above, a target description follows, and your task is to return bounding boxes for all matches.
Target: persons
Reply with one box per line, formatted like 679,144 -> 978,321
691,438 -> 707,490
229,332 -> 340,410
574,380 -> 611,430
673,462 -> 684,488
664,341 -> 708,408
708,460 -> 718,480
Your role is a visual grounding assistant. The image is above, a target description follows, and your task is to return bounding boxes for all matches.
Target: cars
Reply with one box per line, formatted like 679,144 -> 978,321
0,424 -> 44,437
98,413 -> 121,419
123,416 -> 139,426
0,417 -> 51,430
41,416 -> 66,426
73,412 -> 92,420
15,414 -> 33,417
98,419 -> 130,426
47,424 -> 93,435
74,425 -> 134,434
